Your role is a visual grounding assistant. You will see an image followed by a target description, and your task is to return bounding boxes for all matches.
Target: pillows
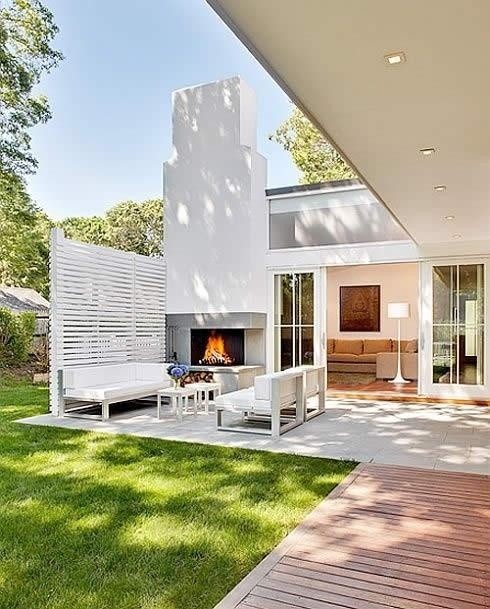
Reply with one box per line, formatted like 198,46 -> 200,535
364,340 -> 391,353
334,340 -> 363,354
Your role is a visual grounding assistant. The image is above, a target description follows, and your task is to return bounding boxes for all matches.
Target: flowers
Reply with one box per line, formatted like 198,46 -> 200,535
167,365 -> 189,379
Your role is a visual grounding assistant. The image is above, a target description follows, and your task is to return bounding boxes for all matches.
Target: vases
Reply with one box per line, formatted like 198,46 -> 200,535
173,380 -> 181,389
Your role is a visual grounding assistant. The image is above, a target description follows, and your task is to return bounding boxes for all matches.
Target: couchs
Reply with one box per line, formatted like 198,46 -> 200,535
214,365 -> 326,438
58,362 -> 173,421
327,339 -> 418,381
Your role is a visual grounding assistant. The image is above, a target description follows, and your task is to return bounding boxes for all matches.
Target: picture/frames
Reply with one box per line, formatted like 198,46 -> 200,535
340,285 -> 380,331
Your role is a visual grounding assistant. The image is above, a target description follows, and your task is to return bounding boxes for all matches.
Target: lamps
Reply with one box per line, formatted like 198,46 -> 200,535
387,302 -> 411,384
